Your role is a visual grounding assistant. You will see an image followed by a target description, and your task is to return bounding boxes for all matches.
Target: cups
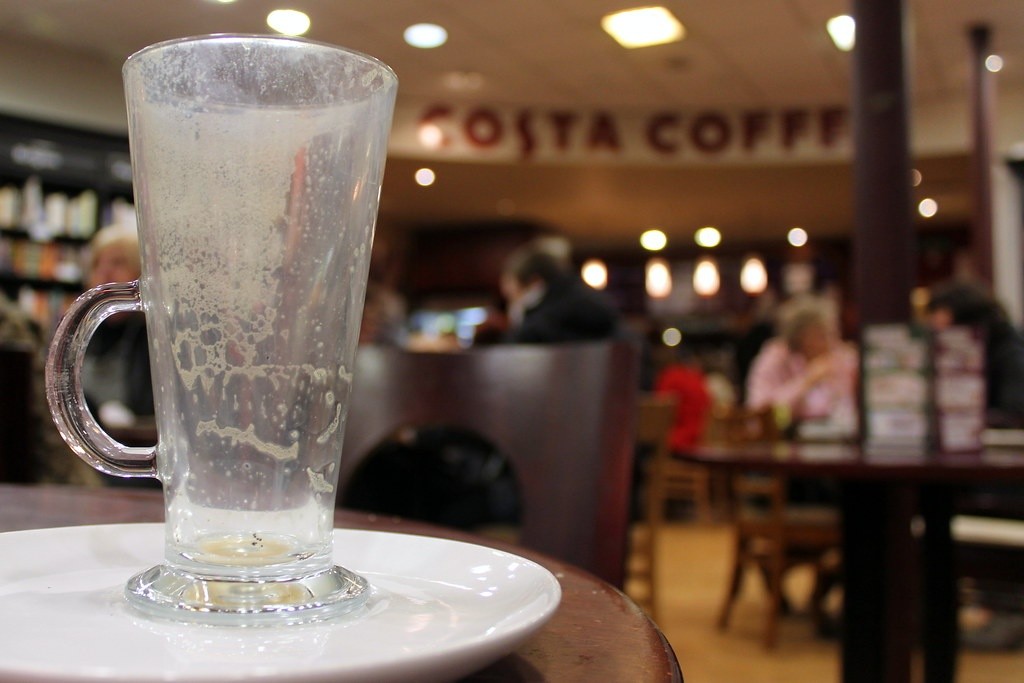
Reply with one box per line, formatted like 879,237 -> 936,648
45,32 -> 397,627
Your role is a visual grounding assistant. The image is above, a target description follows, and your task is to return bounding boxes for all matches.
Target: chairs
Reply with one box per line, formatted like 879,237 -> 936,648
352,340 -> 630,596
625,398 -> 841,651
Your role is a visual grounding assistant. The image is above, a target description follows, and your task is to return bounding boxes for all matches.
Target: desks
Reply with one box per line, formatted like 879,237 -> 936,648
0,482 -> 687,682
672,428 -> 1023,683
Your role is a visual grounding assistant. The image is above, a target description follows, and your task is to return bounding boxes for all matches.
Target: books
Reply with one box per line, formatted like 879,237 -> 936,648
0,179 -> 139,330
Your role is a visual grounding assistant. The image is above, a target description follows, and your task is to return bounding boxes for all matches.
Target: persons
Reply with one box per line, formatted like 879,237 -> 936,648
734,263 -> 1024,618
339,251 -> 733,541
0,294 -> 47,485
63,224 -> 153,425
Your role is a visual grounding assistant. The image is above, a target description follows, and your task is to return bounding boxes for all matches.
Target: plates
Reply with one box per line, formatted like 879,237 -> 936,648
0,523 -> 561,683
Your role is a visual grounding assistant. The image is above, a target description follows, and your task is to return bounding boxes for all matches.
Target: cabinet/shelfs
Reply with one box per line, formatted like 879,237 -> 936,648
0,111 -> 137,293
